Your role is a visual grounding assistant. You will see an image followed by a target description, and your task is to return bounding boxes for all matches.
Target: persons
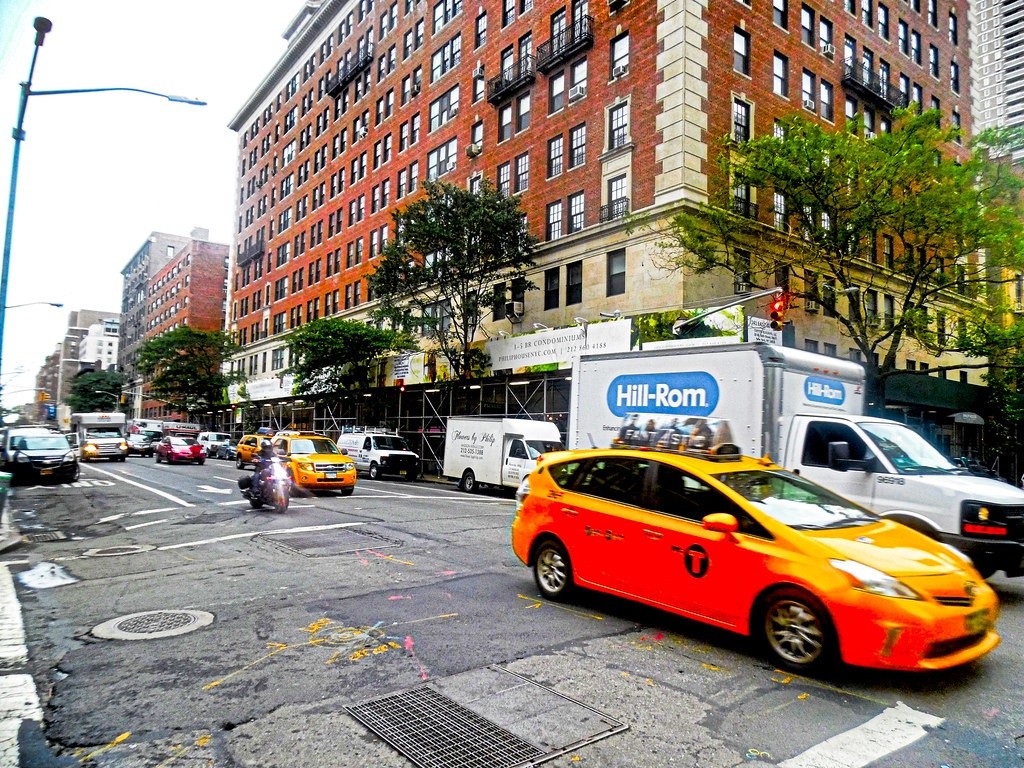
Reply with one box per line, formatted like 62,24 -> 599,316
737,513 -> 752,531
587,471 -> 630,504
248,439 -> 296,500
660,471 -> 699,521
638,466 -> 653,490
619,413 -> 737,461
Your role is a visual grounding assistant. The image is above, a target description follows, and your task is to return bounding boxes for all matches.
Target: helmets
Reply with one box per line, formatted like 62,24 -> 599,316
261,440 -> 273,451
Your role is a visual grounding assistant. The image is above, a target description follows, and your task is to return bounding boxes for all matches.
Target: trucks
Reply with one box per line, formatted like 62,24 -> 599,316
564,342 -> 1024,583
442,415 -> 564,493
336,426 -> 421,483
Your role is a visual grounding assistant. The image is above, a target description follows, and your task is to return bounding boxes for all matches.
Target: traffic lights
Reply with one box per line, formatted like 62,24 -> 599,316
769,299 -> 784,334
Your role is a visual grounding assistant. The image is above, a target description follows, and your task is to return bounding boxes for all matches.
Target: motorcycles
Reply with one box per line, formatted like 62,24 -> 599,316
238,451 -> 293,513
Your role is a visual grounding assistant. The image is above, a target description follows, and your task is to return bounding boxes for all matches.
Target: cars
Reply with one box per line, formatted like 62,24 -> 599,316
215,438 -> 240,459
236,426 -> 274,469
0,410 -> 201,470
156,436 -> 206,465
270,430 -> 357,495
198,431 -> 231,457
12,434 -> 80,483
950,456 -> 1007,483
512,429 -> 1000,681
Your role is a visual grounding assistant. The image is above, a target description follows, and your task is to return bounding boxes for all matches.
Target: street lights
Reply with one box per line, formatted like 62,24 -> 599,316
94,390 -> 118,413
0,13 -> 205,378
